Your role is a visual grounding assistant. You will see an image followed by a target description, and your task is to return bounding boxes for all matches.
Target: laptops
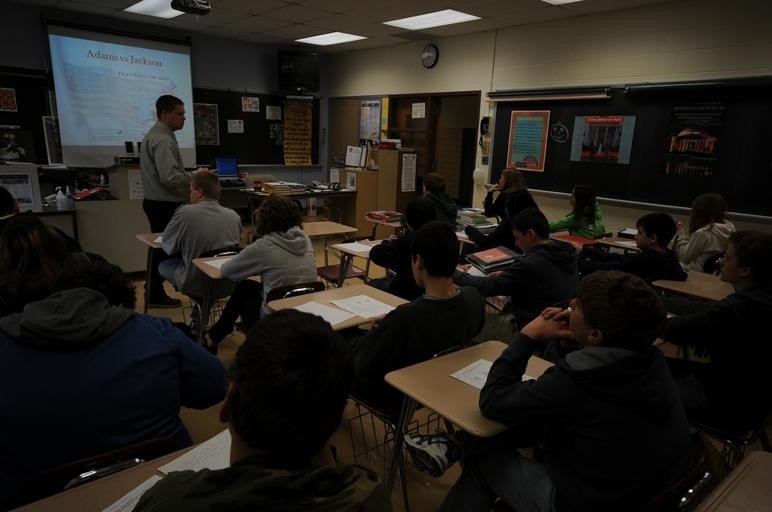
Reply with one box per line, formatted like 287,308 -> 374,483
214,156 -> 247,188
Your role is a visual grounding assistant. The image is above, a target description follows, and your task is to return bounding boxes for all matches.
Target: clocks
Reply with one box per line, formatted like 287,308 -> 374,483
421,44 -> 439,68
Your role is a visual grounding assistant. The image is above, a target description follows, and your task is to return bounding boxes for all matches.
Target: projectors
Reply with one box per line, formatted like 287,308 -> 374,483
171,0 -> 211,15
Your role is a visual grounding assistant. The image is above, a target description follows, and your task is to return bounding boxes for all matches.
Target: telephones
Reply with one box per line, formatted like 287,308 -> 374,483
480,117 -> 489,135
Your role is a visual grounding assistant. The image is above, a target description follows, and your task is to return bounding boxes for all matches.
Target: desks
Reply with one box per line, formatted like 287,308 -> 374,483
384,339 -> 559,506
19,444 -> 203,511
88,182 -> 110,187
34,206 -> 80,241
133,211 -> 733,359
239,186 -> 358,244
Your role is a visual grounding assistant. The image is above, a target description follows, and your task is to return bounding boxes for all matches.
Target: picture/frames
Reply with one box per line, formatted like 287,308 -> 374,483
0,164 -> 43,213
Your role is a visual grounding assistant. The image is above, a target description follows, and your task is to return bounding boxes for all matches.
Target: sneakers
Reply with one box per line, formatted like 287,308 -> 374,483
144,289 -> 182,307
404,431 -> 462,478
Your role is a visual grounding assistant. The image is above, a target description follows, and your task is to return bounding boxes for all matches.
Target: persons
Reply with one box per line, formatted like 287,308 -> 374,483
453,208 -> 579,348
1,253 -> 229,511
676,193 -> 735,273
548,184 -> 606,239
569,213 -> 687,285
203,194 -> 317,356
140,95 -> 219,308
159,167 -> 243,299
0,187 -> 84,256
400,172 -> 456,232
1,214 -> 133,310
338,221 -> 487,416
457,189 -> 538,261
484,168 -> 528,218
369,196 -> 436,300
400,270 -> 696,510
660,229 -> 772,441
133,311 -> 394,511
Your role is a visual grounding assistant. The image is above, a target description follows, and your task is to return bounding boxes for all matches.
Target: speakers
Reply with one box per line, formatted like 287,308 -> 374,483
125,142 -> 136,157
138,142 -> 142,153
276,49 -> 320,93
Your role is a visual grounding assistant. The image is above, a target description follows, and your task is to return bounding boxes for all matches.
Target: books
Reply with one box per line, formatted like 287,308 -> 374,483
455,204 -> 500,235
263,180 -> 293,197
368,210 -> 405,224
466,243 -> 520,271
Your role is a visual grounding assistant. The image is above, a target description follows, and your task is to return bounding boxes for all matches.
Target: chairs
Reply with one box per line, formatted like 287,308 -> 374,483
340,344 -> 469,505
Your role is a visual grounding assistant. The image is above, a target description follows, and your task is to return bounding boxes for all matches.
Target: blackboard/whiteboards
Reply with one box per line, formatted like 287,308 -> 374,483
42,116 -> 60,166
0,71 -> 62,165
487,73 -> 771,219
193,86 -> 324,167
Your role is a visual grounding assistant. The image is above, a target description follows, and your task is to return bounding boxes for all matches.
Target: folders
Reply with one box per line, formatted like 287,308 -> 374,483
464,243 -> 526,276
